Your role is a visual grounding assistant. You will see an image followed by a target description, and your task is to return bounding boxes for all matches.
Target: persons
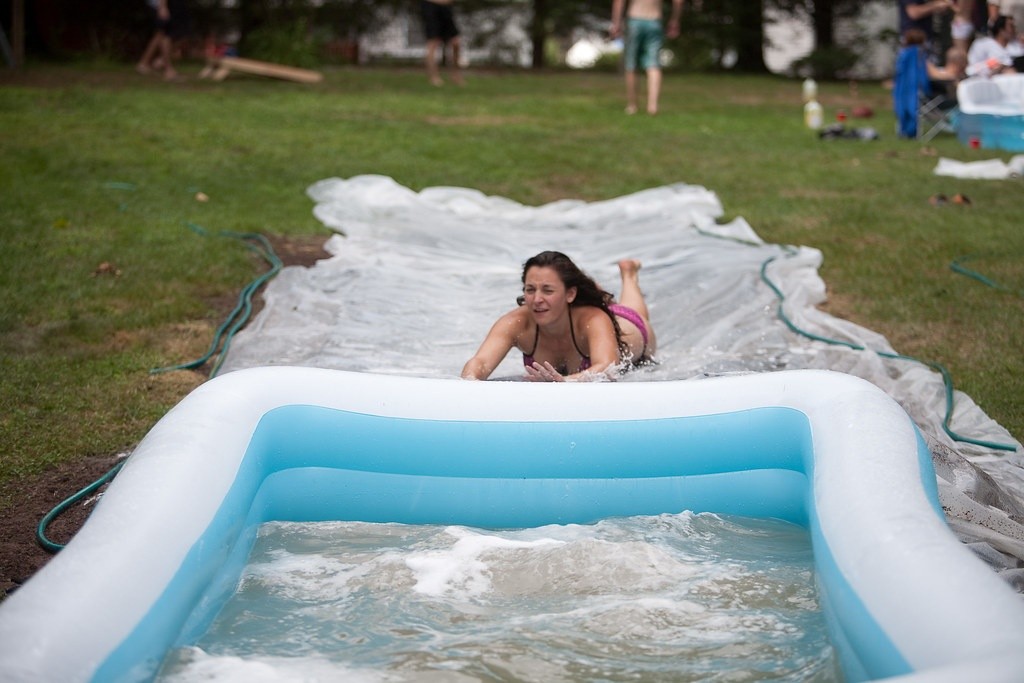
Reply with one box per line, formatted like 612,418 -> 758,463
895,0 -> 1024,113
607,0 -> 683,116
415,0 -> 468,88
135,0 -> 188,81
198,0 -> 247,81
460,251 -> 657,381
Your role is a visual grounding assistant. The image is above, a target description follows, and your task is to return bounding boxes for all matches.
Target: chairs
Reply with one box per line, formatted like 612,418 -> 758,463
916,89 -> 959,143
